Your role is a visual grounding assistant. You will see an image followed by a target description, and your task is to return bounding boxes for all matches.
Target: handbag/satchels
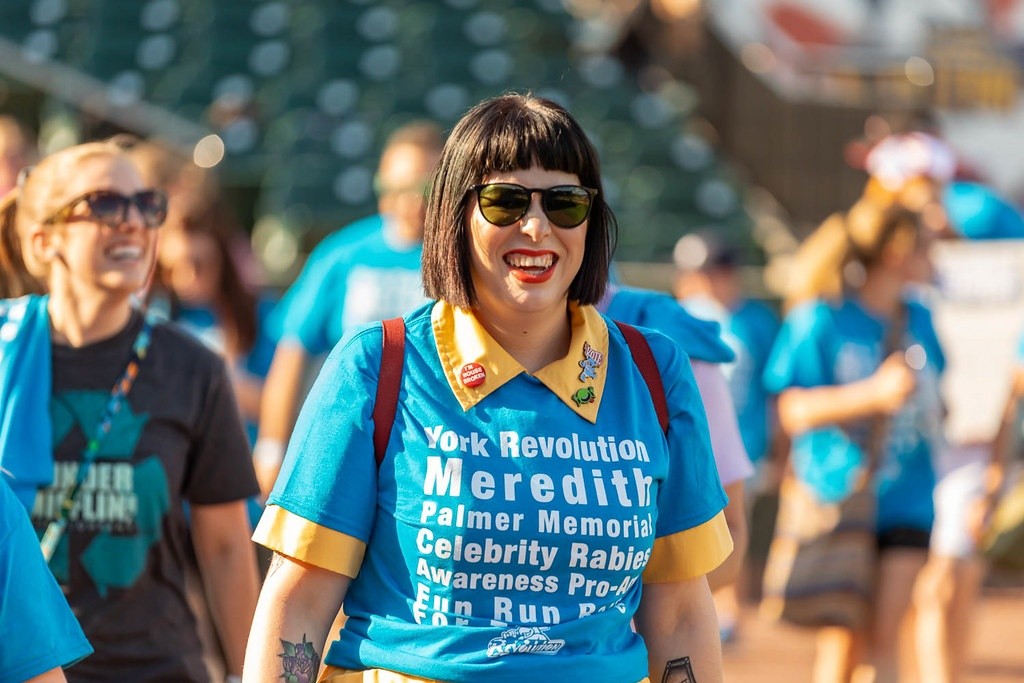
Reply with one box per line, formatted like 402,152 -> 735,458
732,468 -> 876,629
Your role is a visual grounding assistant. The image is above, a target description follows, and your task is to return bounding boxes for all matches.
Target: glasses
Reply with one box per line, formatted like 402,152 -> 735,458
41,188 -> 168,228
469,183 -> 598,229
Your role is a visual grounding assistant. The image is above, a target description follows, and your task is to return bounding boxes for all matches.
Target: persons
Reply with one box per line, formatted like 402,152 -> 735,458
239,93 -> 736,683
0,110 -> 444,683
597,136 -> 1024,683
